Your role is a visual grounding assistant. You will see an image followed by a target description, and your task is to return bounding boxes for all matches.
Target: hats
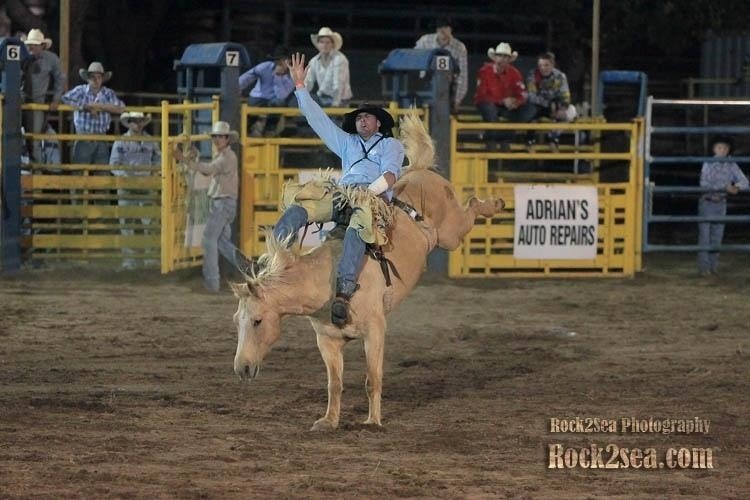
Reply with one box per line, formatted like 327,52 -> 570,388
310,27 -> 342,51
23,29 -> 52,49
120,112 -> 151,128
488,43 -> 517,63
428,17 -> 456,31
708,135 -> 735,154
342,105 -> 393,133
79,62 -> 112,81
263,47 -> 292,59
208,122 -> 238,145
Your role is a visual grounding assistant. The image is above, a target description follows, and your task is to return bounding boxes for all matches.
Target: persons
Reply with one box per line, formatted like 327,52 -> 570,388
169,118 -> 261,297
1,0 -> 571,278
232,49 -> 407,326
696,134 -> 750,278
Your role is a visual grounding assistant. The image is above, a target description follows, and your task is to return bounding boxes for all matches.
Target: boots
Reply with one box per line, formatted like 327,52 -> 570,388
705,197 -> 725,202
331,277 -> 356,324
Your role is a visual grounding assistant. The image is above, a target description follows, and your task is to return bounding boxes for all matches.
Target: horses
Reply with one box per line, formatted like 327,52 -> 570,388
225,96 -> 506,433
